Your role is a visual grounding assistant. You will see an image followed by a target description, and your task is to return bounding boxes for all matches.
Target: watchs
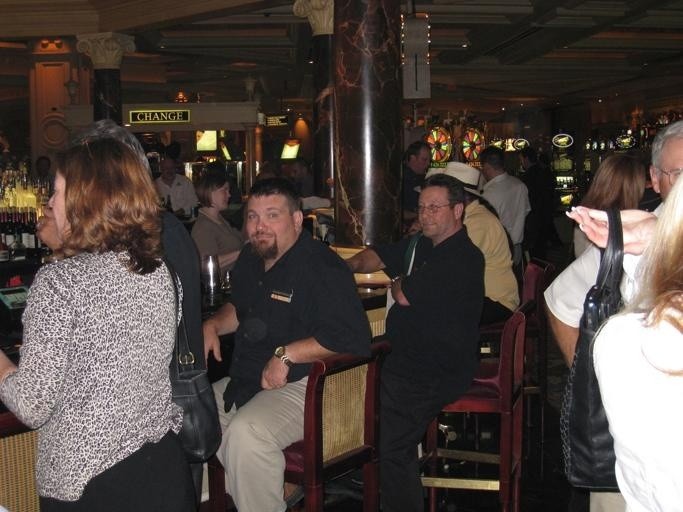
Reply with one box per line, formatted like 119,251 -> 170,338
275,345 -> 295,368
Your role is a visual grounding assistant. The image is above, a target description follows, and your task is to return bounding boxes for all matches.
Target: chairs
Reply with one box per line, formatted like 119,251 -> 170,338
421,311 -> 528,509
203,340 -> 380,511
482,260 -> 551,445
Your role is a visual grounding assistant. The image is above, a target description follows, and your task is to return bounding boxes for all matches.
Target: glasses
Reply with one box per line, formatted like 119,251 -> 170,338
414,203 -> 453,213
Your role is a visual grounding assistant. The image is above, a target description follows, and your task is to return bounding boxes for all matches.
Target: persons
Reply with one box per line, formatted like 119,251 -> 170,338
402,141 -> 557,328
592,177 -> 682,512
344,174 -> 486,510
543,121 -> 683,511
155,157 -> 332,373
202,177 -> 373,511
2,120 -> 202,509
36,156 -> 49,178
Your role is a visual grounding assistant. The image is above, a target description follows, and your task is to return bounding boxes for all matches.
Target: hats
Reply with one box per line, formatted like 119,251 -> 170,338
429,162 -> 482,197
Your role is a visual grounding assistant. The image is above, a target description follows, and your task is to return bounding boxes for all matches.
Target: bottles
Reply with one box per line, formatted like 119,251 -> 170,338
200,254 -> 218,295
207,296 -> 217,316
222,269 -> 232,296
0,169 -> 51,261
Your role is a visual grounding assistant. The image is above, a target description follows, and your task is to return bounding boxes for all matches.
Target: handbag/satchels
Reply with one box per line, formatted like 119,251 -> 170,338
559,285 -> 625,492
170,355 -> 223,463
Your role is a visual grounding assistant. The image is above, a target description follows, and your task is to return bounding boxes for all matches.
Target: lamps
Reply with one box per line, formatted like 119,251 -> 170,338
175,87 -> 189,102
280,130 -> 301,160
64,80 -> 80,105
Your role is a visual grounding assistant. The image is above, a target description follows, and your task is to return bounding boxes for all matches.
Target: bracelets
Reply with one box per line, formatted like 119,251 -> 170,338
391,276 -> 399,286
